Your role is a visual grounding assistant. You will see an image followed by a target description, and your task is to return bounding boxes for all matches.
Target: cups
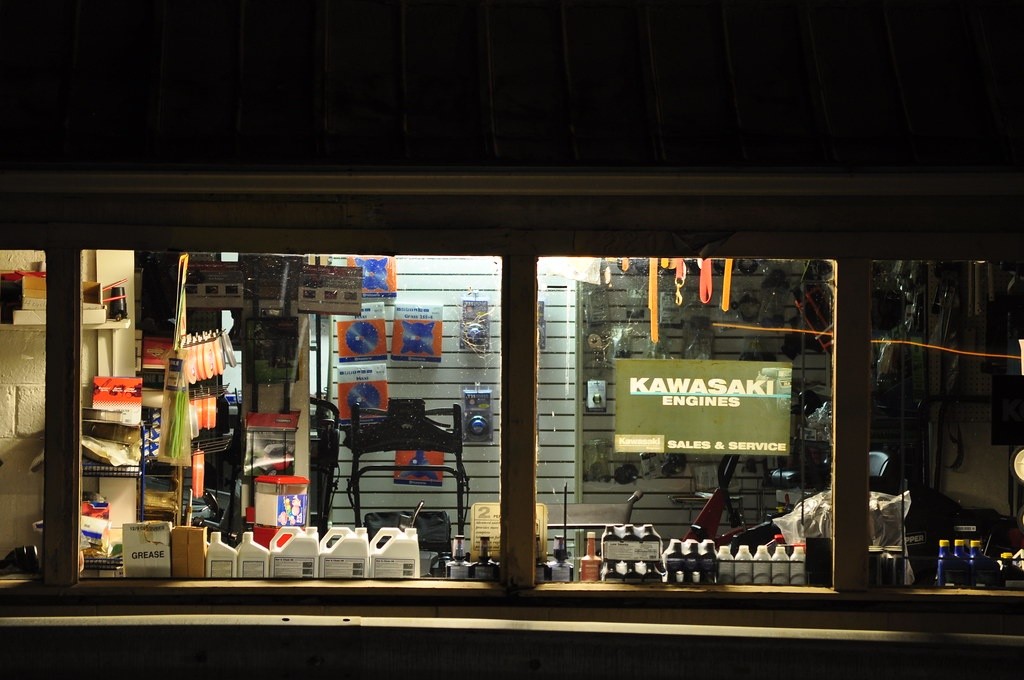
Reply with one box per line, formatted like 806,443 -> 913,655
109,286 -> 128,319
867,545 -> 903,589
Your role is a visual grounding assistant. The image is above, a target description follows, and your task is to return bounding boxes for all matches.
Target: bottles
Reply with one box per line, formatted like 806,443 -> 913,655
753,545 -> 772,584
547,535 -> 574,582
662,538 -> 718,583
716,546 -> 735,584
772,546 -> 790,585
445,535 -> 471,579
937,538 -> 1001,590
790,546 -> 806,585
735,545 -> 753,584
471,536 -> 500,579
601,523 -> 666,583
270,526 -> 421,580
580,531 -> 602,580
205,531 -> 270,578
1000,552 -> 1024,591
534,533 -> 552,582
